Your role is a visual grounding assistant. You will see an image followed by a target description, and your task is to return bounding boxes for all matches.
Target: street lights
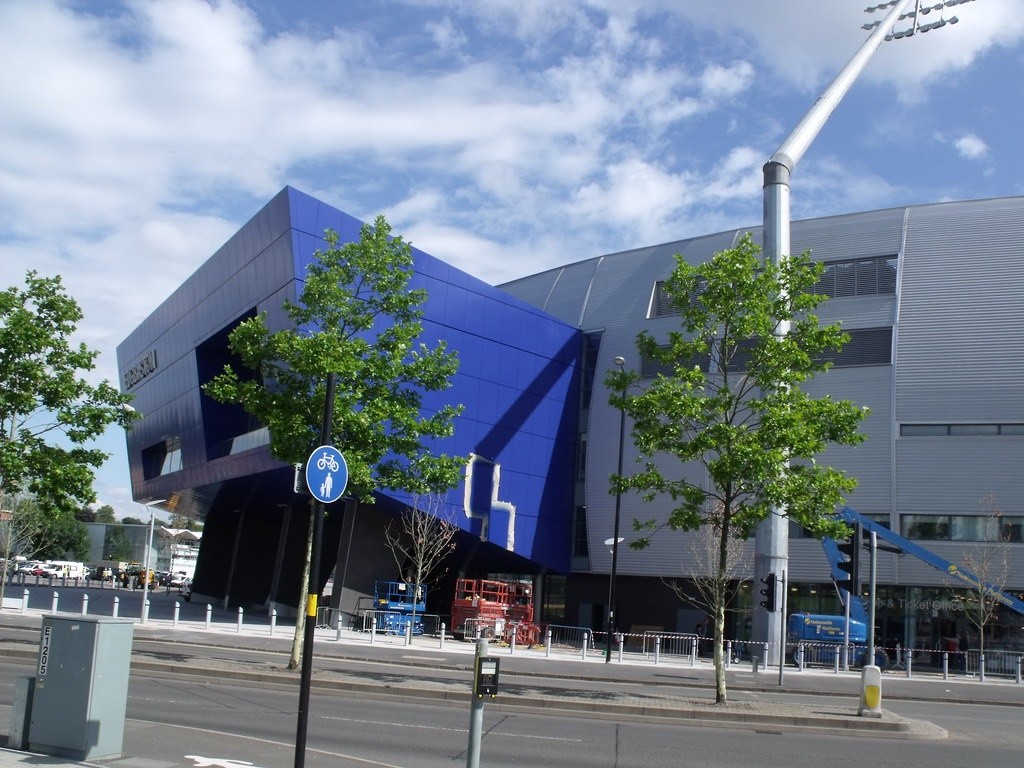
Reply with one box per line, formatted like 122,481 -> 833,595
752,0 -> 978,670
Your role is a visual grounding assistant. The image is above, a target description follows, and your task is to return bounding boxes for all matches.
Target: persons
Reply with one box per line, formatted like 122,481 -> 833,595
943,633 -> 970,671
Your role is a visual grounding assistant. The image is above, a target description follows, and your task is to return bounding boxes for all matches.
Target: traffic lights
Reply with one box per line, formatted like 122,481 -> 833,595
836,522 -> 864,597
758,572 -> 778,615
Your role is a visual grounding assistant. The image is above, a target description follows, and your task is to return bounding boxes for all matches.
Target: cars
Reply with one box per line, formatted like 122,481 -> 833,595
88,558 -> 194,594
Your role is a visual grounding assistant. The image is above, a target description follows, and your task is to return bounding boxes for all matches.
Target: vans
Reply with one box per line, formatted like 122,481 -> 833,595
12,556 -> 27,575
20,563 -> 43,576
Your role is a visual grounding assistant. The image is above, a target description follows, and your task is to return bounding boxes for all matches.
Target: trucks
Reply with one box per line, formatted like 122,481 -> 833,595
43,561 -> 83,580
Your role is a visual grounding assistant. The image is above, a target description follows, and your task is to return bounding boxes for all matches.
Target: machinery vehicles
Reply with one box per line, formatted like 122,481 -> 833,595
787,507 -> 1024,670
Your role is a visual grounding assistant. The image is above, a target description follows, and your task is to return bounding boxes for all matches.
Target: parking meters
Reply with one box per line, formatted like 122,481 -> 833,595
466,626 -> 501,768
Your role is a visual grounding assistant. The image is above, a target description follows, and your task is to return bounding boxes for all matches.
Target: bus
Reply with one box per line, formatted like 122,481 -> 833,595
451,577 -> 534,643
354,580 -> 428,636
